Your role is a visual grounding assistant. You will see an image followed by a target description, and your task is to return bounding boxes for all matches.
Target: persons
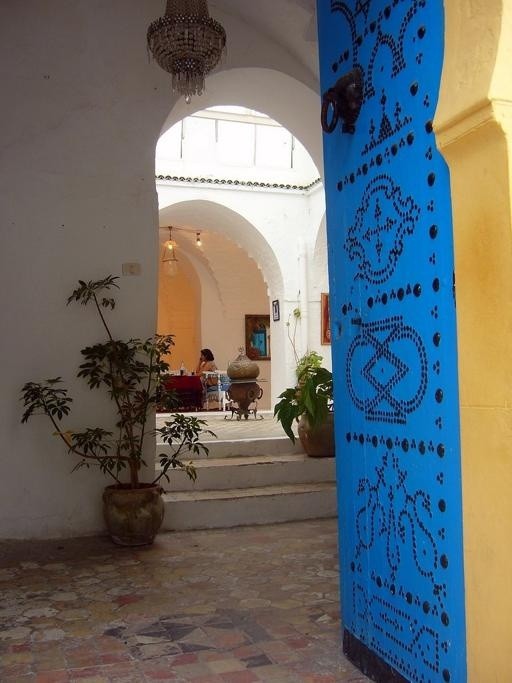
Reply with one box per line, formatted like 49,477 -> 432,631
195,348 -> 219,410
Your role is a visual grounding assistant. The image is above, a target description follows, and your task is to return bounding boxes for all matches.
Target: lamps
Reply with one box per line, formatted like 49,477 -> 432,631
146,0 -> 228,104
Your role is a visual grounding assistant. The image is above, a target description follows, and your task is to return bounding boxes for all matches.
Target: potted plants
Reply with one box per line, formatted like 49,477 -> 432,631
273,363 -> 335,457
22,272 -> 217,549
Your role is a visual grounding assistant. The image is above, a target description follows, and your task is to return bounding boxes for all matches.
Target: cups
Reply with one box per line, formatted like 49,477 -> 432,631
191,368 -> 196,375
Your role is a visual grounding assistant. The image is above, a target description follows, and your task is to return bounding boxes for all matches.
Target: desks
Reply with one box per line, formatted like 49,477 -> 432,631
158,373 -> 206,411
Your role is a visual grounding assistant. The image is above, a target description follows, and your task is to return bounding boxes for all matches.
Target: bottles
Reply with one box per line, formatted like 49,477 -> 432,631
180,360 -> 185,375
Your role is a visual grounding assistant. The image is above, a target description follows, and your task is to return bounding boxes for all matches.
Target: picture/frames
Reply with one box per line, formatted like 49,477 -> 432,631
245,313 -> 272,360
